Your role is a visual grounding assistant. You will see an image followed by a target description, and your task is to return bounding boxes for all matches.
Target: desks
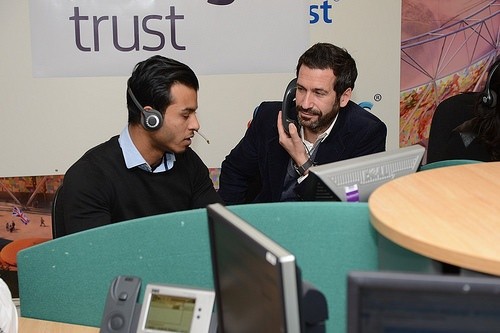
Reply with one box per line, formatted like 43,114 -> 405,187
368,161 -> 500,276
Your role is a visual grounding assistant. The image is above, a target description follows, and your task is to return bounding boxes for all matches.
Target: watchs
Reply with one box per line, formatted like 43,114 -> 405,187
294,159 -> 313,176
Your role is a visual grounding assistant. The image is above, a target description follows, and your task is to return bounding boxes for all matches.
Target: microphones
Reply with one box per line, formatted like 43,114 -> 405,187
196,130 -> 210,144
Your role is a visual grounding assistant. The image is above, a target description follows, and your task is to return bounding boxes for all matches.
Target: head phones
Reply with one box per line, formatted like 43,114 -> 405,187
128,87 -> 163,131
483,63 -> 500,107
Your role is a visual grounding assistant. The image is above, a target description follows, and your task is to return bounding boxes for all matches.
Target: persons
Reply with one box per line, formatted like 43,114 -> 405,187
63,55 -> 226,236
216,43 -> 387,206
445,56 -> 500,162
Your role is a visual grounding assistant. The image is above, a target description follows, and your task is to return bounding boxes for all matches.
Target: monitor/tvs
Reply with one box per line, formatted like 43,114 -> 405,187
306,144 -> 425,203
346,272 -> 500,333
208,203 -> 329,333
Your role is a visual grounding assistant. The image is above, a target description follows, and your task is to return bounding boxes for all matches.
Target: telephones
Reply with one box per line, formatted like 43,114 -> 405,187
101,275 -> 218,333
281,78 -> 298,136
